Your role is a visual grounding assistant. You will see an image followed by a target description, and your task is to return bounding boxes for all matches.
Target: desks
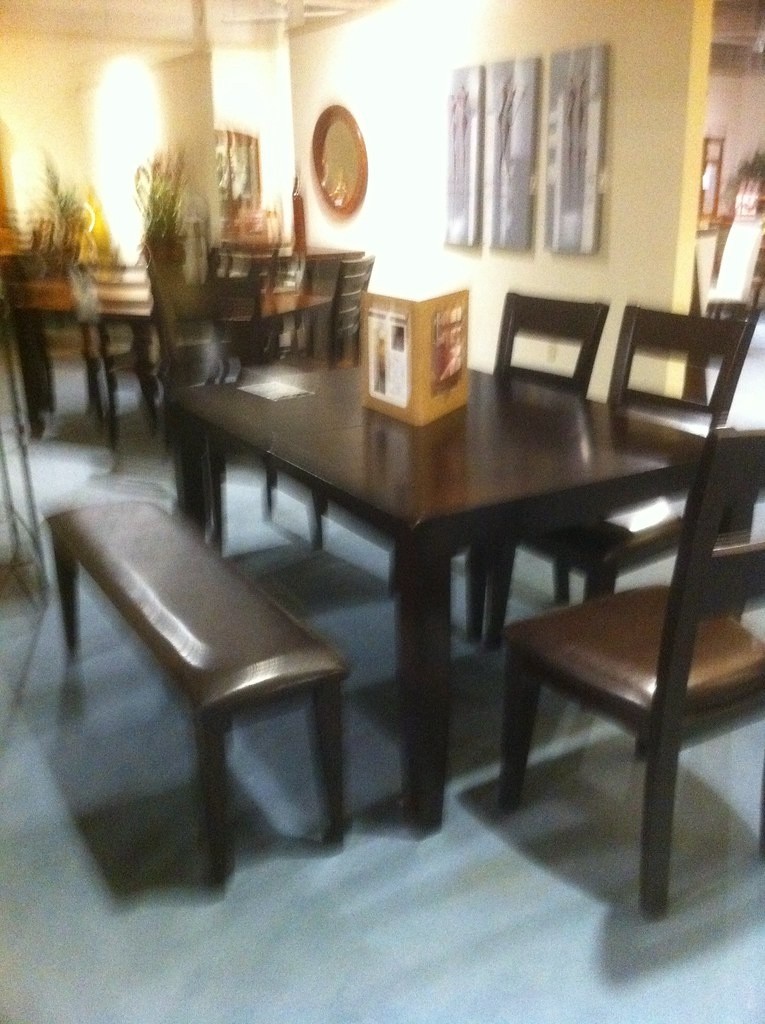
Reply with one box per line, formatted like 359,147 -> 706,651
5,263 -> 333,440
219,243 -> 366,297
175,364 -> 752,835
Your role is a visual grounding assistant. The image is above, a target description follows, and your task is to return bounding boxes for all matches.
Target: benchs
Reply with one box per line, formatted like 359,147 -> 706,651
46,498 -> 349,892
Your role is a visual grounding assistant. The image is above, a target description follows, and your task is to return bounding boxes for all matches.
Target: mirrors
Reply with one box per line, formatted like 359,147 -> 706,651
311,105 -> 369,216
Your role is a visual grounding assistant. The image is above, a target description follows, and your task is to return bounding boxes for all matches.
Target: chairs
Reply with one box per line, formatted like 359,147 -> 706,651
691,220 -> 764,321
388,292 -> 610,642
486,304 -> 761,646
0,194 -> 375,551
499,427 -> 765,918
